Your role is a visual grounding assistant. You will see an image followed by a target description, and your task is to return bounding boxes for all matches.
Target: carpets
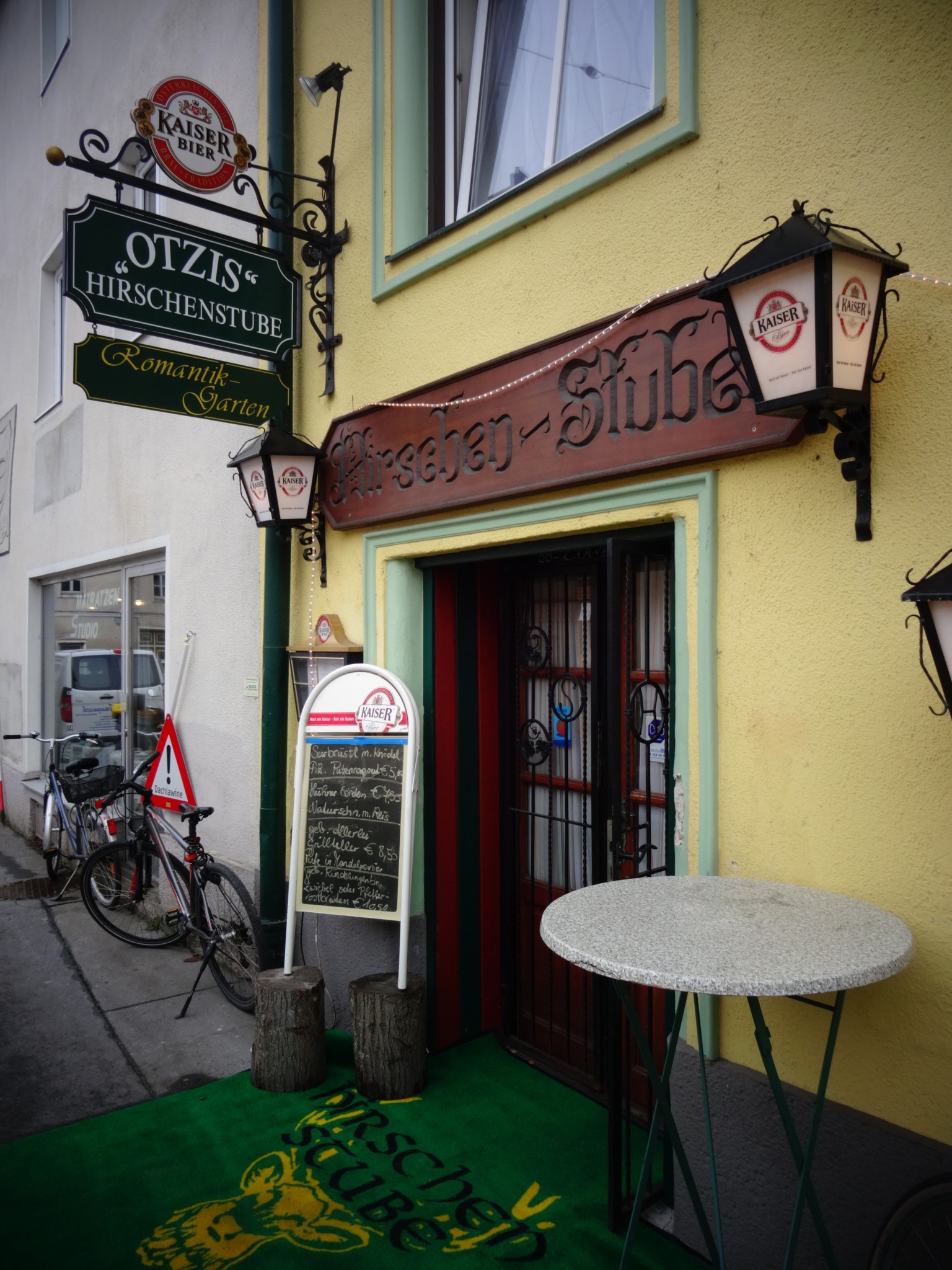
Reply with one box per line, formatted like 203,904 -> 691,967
0,1029 -> 712,1270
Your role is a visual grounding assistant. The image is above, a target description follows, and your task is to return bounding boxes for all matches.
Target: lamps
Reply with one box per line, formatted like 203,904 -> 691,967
300,62 -> 352,185
901,550 -> 952,719
698,198 -> 909,541
227,417 -> 328,587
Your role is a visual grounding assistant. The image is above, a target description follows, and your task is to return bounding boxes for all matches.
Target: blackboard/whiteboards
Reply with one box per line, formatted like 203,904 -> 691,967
287,663 -> 417,921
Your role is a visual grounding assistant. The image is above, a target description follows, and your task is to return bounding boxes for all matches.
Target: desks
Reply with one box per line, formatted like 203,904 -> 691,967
539,875 -> 913,1270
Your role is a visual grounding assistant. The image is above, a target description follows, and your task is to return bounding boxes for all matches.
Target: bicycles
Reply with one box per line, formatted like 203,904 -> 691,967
79,752 -> 274,1022
2,729 -> 123,908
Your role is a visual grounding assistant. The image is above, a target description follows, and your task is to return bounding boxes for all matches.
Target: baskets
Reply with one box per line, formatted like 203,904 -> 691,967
58,765 -> 125,804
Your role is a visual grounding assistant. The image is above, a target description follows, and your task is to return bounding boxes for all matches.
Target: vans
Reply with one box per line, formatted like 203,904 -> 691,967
51,649 -> 164,743
